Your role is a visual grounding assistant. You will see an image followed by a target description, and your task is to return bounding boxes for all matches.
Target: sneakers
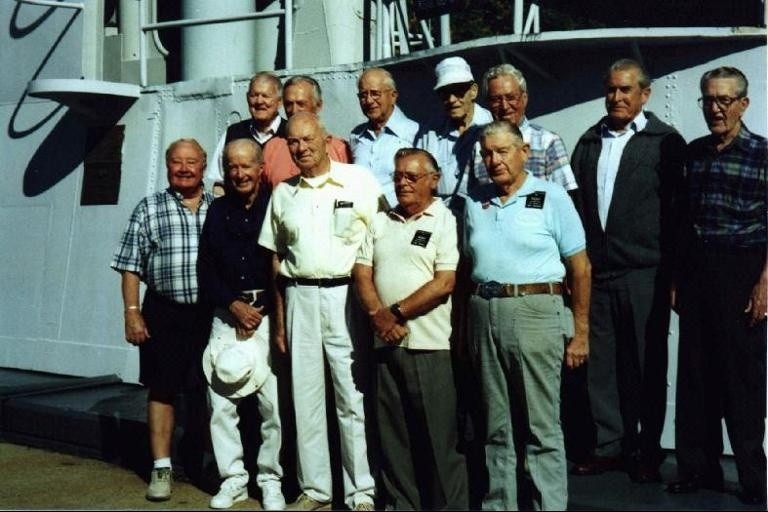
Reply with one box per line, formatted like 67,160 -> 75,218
262,487 -> 286,512
210,487 -> 249,509
286,494 -> 333,512
146,467 -> 174,502
352,503 -> 375,511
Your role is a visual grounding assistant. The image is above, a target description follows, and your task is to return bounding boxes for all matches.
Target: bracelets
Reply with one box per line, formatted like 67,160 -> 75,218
125,304 -> 142,311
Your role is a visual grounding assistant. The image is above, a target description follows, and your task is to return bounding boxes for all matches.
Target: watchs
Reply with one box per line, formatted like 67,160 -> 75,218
391,303 -> 408,322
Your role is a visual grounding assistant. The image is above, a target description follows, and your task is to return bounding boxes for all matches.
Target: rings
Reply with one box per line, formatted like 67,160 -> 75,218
763,311 -> 767,315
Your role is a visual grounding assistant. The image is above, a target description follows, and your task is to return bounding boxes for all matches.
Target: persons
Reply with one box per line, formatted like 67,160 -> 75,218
564,58 -> 688,485
351,147 -> 470,512
261,74 -> 352,195
111,137 -> 222,502
461,119 -> 594,510
254,112 -> 391,510
413,56 -> 496,442
666,66 -> 767,506
349,68 -> 420,211
193,139 -> 288,510
201,71 -> 288,203
455,64 -> 580,473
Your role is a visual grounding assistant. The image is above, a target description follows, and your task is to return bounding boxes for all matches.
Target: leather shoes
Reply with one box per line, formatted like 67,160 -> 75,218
667,471 -> 723,494
572,453 -> 616,476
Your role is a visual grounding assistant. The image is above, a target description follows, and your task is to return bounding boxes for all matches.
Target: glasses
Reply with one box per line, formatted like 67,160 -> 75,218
486,92 -> 524,106
356,88 -> 395,100
698,95 -> 744,110
389,171 -> 434,183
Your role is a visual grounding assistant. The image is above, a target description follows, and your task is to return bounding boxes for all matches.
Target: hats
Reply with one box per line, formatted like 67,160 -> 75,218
202,327 -> 272,399
433,56 -> 474,96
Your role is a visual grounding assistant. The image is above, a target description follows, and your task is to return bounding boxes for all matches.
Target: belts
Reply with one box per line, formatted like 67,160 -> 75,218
146,292 -> 185,317
286,277 -> 353,288
472,281 -> 564,300
235,291 -> 267,302
